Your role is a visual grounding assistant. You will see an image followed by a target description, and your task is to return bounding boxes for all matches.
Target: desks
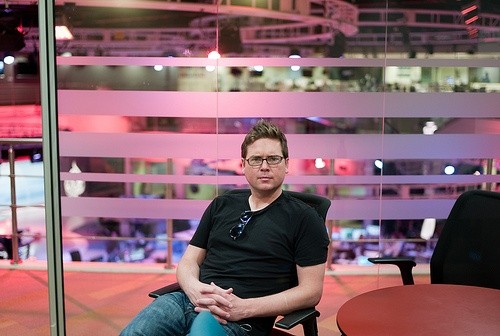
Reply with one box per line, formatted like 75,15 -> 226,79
335,284 -> 500,336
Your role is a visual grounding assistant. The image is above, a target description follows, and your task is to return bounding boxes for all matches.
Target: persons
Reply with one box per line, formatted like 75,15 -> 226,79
119,121 -> 330,336
370,186 -> 424,241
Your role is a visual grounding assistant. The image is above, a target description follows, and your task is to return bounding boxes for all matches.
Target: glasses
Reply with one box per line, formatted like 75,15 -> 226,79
244,154 -> 286,166
229,210 -> 255,241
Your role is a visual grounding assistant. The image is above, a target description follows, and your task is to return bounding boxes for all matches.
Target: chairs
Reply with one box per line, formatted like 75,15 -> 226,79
148,189 -> 331,336
368,189 -> 500,291
